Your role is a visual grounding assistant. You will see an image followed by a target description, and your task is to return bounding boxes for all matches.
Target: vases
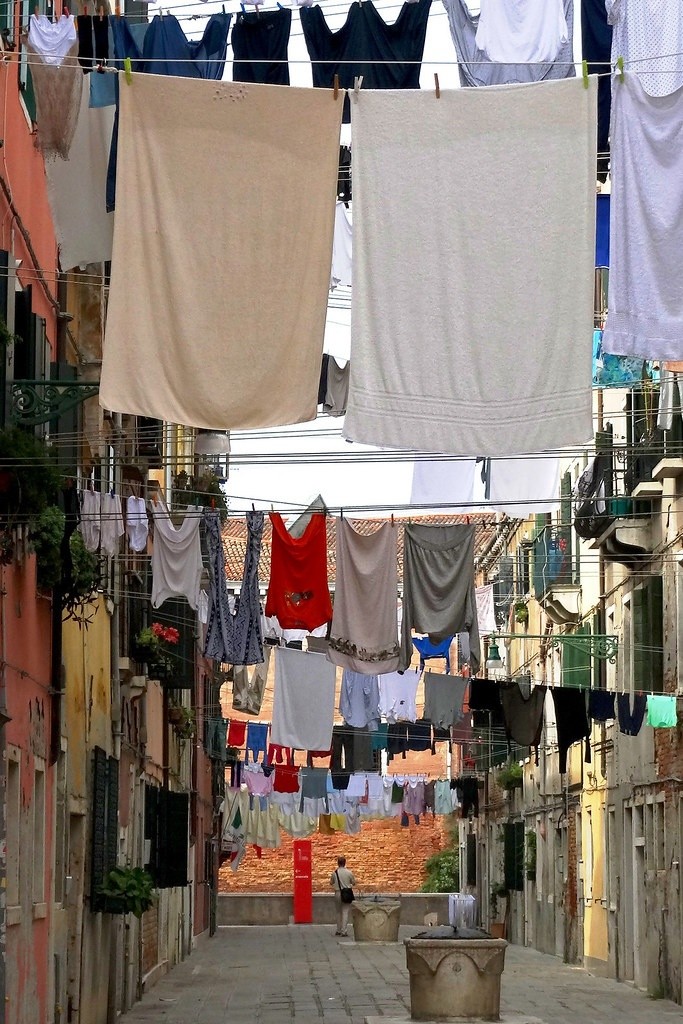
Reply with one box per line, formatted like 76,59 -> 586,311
131,647 -> 154,662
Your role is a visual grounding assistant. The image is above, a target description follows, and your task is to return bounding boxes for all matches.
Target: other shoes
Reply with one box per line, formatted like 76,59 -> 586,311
335,932 -> 341,935
342,934 -> 347,937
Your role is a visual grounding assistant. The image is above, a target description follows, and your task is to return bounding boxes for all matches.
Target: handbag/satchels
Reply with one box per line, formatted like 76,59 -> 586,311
341,888 -> 355,903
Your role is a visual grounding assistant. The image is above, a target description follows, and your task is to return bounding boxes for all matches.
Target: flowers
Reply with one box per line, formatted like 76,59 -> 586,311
136,623 -> 179,647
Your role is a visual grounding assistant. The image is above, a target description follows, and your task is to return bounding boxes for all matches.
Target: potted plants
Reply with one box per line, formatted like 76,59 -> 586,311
90,866 -> 154,919
514,596 -> 529,623
524,830 -> 537,880
490,880 -> 506,938
226,746 -> 241,756
168,703 -> 195,739
496,758 -> 524,789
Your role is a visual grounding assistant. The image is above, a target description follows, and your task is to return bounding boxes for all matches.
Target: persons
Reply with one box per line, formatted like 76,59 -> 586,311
330,857 -> 355,937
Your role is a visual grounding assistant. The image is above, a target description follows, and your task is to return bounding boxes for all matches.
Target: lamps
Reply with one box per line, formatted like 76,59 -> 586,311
485,631 -> 621,667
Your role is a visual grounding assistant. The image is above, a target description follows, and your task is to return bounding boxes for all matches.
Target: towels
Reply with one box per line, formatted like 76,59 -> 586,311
269,645 -> 337,752
344,72 -> 599,457
99,68 -> 347,433
600,70 -> 683,363
